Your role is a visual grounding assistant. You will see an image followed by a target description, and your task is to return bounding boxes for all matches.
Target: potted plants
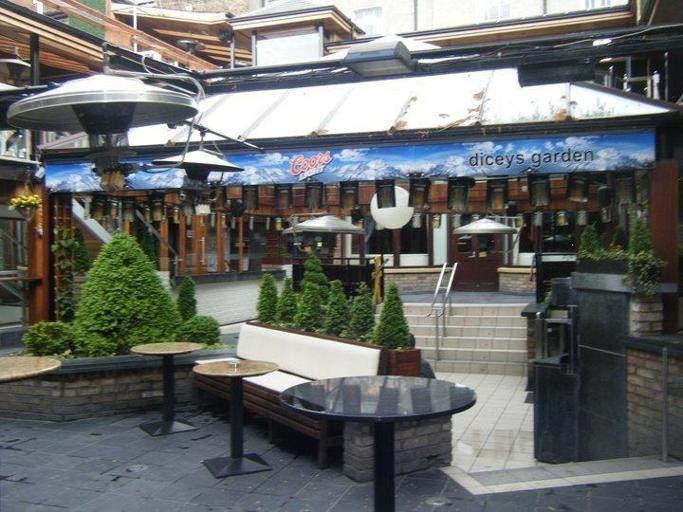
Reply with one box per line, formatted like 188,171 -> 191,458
247,250 -> 422,377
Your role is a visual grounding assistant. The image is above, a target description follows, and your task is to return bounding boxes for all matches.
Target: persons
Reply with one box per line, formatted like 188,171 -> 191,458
357,205 -> 377,254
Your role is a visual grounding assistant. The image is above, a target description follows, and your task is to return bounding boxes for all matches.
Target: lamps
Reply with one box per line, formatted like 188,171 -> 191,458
176,38 -> 206,52
451,215 -> 517,235
280,212 -> 365,245
150,132 -> 245,203
0,55 -> 31,82
5,41 -> 200,173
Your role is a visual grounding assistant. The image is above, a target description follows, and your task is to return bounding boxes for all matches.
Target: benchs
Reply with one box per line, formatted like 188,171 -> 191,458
192,320 -> 388,469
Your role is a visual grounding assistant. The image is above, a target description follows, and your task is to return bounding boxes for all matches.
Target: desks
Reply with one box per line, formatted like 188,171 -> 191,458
130,342 -> 205,436
278,375 -> 477,512
192,360 -> 280,479
0,356 -> 62,384
